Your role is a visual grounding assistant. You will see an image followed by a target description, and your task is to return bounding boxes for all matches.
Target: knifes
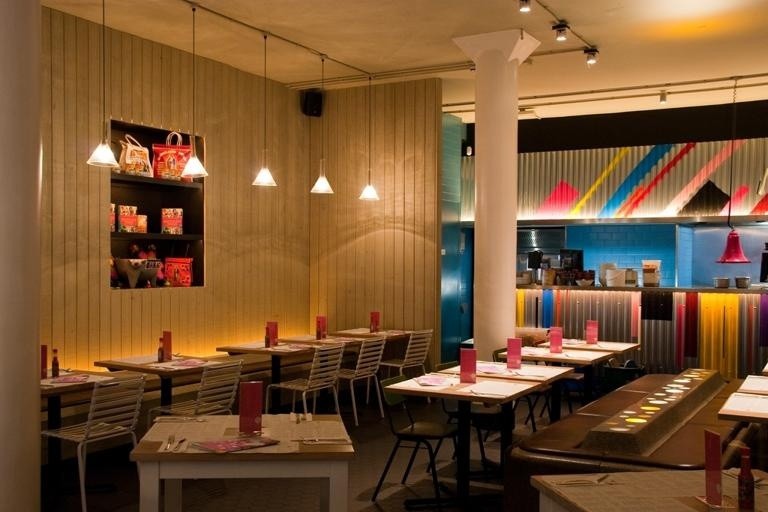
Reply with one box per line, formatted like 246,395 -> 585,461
557,481 -> 626,486
168,437 -> 187,453
290,438 -> 347,442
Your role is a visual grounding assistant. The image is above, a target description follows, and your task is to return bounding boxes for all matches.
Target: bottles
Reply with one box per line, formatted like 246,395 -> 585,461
316,321 -> 321,339
52,349 -> 59,378
158,338 -> 163,363
370,315 -> 374,333
265,327 -> 270,347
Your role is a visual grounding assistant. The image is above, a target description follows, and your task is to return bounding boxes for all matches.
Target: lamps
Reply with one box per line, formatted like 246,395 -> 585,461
180,6 -> 210,180
88,0 -> 121,171
309,56 -> 334,195
358,76 -> 381,201
584,47 -> 599,65
250,34 -> 279,187
548,21 -> 573,44
519,0 -> 533,15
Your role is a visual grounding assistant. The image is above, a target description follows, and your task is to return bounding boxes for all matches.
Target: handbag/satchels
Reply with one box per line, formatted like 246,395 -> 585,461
117,133 -> 154,178
151,131 -> 195,183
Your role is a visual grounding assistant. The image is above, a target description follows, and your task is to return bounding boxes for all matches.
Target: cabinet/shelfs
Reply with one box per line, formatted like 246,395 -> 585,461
107,118 -> 206,289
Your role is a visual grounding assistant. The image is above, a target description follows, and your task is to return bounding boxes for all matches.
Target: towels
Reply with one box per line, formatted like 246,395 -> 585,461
288,412 -> 313,423
549,474 -> 627,486
152,415 -> 207,423
156,439 -> 190,454
301,436 -> 353,445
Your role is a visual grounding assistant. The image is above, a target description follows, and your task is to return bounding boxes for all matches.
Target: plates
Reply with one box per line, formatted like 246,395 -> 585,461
575,280 -> 595,286
516,277 -> 530,285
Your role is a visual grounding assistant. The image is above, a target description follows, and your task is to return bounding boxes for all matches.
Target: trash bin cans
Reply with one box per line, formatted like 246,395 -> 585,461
604,362 -> 644,395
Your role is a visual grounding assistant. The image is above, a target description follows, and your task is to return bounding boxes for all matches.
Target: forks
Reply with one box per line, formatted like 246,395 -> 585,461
470,389 -> 505,396
164,433 -> 176,451
508,370 -> 545,378
554,474 -> 610,484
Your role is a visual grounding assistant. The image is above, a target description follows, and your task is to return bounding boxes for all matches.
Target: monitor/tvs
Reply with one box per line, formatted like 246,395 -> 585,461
559,249 -> 584,269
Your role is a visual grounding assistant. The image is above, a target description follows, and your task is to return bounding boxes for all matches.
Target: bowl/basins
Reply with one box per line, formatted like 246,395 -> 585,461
714,278 -> 730,289
735,277 -> 751,288
751,284 -> 761,290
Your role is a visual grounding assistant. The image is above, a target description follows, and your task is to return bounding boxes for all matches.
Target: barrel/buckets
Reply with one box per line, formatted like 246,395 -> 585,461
606,270 -> 625,286
642,260 -> 660,287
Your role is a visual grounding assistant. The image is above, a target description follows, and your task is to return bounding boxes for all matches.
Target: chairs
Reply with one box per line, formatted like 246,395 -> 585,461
379,326 -> 432,405
426,362 -> 512,473
338,336 -> 385,426
40,375 -> 142,512
370,376 -> 462,504
148,359 -> 245,419
493,343 -> 557,432
264,340 -> 341,418
